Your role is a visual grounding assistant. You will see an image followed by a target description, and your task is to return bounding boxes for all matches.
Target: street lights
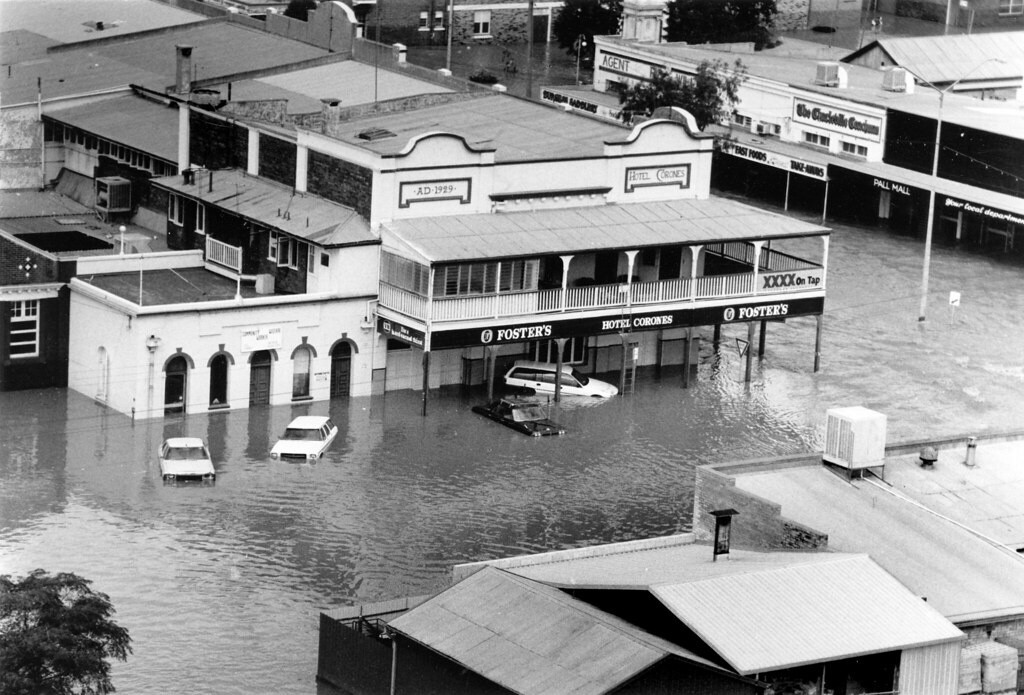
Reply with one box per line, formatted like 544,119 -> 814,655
575,30 -> 586,85
875,51 -> 1005,320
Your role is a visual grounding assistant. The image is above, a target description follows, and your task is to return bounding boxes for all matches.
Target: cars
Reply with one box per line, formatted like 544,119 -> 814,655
158,437 -> 215,486
500,358 -> 619,401
267,416 -> 340,464
479,402 -> 567,436
